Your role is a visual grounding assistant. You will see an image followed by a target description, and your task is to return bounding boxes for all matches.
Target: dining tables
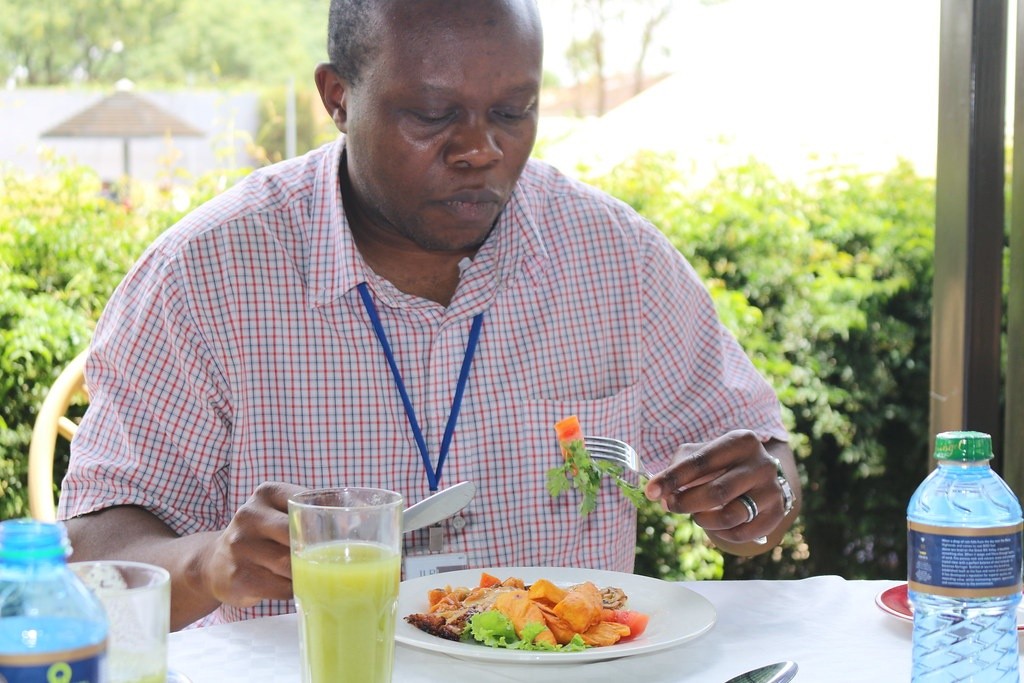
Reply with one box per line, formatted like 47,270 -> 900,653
169,572 -> 914,683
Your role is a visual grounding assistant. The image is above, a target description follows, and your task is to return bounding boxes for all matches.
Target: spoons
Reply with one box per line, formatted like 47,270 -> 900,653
725,661 -> 800,683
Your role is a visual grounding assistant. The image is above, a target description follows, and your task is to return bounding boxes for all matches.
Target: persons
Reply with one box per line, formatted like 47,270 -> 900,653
61,1 -> 802,636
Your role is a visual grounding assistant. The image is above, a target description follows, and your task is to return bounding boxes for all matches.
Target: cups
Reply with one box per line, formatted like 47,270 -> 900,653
285,488 -> 401,683
61,560 -> 172,683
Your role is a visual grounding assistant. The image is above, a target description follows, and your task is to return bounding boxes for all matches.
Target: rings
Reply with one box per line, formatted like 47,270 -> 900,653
738,495 -> 758,524
751,536 -> 769,546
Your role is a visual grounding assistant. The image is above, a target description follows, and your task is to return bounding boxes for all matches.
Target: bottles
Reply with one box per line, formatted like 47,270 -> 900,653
0,516 -> 112,683
905,430 -> 1024,682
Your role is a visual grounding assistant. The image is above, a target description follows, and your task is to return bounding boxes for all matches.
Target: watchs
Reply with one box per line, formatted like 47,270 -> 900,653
774,458 -> 797,517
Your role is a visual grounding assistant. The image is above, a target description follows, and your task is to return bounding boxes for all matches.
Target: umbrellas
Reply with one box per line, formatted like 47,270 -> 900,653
40,89 -> 207,205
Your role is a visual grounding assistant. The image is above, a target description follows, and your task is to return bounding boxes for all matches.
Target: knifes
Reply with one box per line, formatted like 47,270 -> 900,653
401,480 -> 477,539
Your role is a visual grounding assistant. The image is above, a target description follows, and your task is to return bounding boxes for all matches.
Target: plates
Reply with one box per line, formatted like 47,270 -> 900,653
388,565 -> 717,666
874,582 -> 1024,633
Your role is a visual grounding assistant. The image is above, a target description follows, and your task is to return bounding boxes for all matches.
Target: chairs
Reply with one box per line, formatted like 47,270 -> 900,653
27,345 -> 91,524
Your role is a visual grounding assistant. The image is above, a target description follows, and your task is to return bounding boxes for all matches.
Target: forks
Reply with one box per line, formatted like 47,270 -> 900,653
580,436 -> 769,546
